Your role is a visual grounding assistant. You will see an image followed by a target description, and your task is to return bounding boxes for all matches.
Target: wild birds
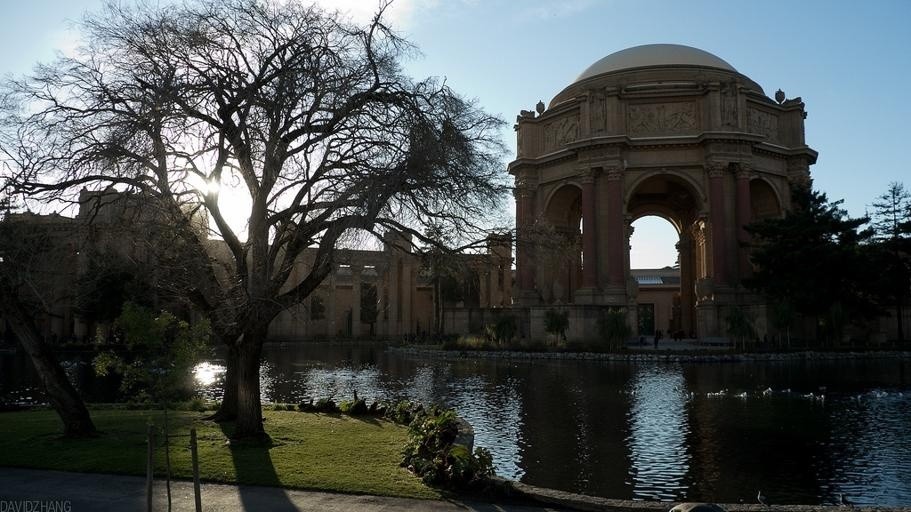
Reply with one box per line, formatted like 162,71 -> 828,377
839,492 -> 855,510
756,490 -> 771,509
